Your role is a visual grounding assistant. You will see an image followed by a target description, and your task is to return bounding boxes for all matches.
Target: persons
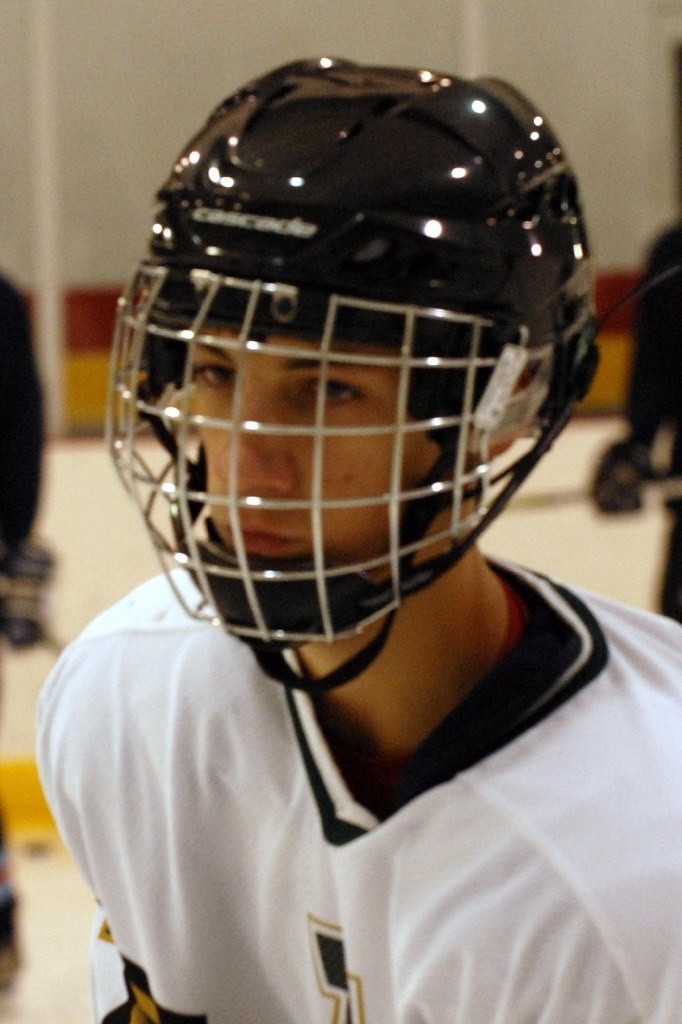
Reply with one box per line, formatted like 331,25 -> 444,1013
588,218 -> 681,624
1,270 -> 61,996
38,50 -> 681,1024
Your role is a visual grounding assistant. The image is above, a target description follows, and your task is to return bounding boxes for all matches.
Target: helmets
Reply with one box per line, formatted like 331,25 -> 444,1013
105,55 -> 597,643
